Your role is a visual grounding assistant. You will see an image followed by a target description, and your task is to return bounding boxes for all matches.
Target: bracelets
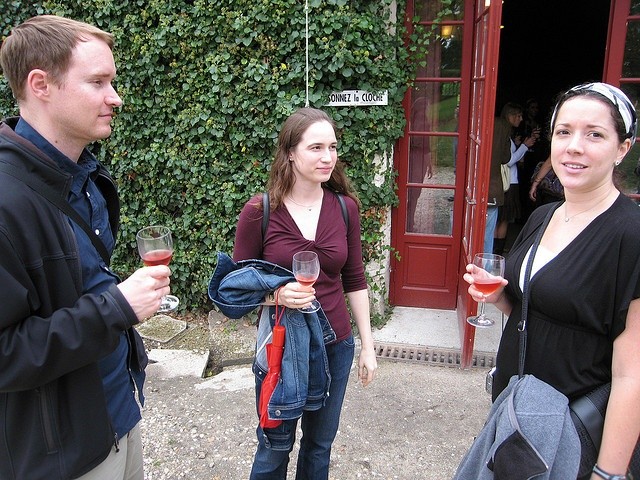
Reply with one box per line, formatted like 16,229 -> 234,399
591,466 -> 624,478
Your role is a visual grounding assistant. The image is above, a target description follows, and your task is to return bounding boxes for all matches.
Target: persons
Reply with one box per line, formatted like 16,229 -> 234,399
463,81 -> 639,479
2,13 -> 170,475
525,97 -> 542,141
528,153 -> 553,202
483,118 -> 512,272
231,106 -> 379,479
494,102 -> 536,256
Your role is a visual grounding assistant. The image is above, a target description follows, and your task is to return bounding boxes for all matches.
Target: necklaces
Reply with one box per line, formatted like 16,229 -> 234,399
283,187 -> 324,212
563,184 -> 616,222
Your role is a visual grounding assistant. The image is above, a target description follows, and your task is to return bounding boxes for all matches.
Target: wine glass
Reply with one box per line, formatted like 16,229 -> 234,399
294,250 -> 321,313
468,251 -> 505,328
134,224 -> 180,316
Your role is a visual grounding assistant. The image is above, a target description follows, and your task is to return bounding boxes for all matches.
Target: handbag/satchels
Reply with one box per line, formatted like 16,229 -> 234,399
516,199 -> 640,479
